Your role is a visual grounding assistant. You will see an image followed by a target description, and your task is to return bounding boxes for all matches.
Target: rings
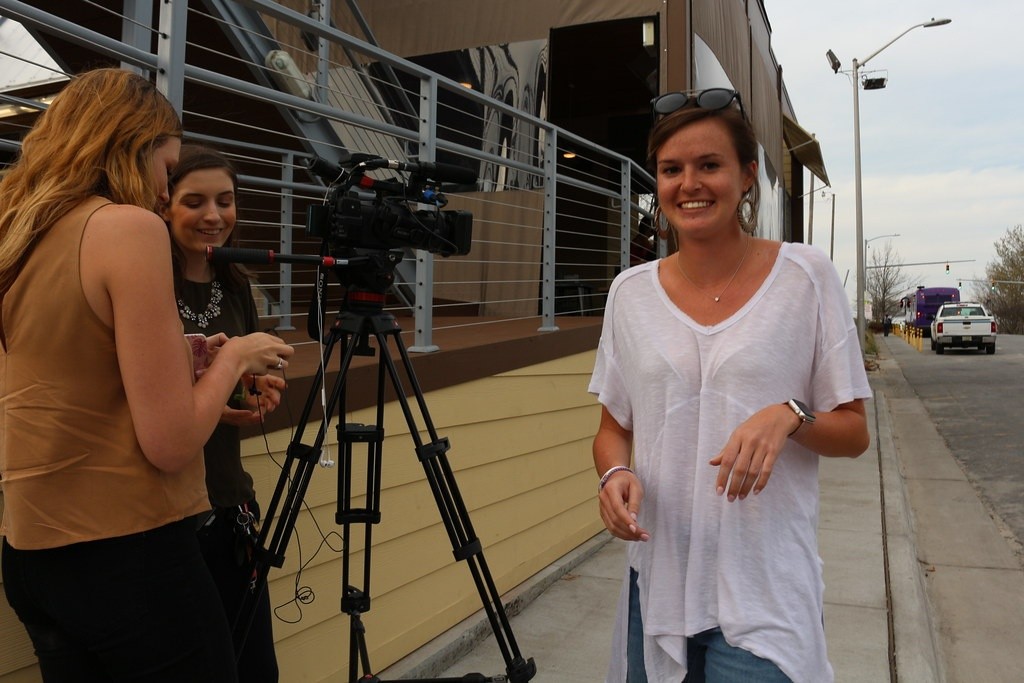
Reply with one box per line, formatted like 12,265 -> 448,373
276,359 -> 284,370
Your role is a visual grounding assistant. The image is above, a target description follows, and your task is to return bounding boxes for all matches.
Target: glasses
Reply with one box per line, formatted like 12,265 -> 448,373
649,88 -> 745,128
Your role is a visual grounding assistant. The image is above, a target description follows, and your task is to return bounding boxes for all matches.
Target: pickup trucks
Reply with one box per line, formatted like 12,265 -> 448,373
926,301 -> 997,354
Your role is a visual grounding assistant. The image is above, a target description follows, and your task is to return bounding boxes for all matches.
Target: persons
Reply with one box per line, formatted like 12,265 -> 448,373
155,145 -> 286,683
0,69 -> 294,682
584,88 -> 875,682
630,216 -> 655,264
882,314 -> 893,336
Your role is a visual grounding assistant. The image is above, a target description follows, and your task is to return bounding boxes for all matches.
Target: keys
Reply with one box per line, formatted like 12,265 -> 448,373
252,520 -> 261,534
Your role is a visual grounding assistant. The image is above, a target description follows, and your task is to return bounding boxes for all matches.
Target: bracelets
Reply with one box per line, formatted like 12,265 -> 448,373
598,466 -> 634,492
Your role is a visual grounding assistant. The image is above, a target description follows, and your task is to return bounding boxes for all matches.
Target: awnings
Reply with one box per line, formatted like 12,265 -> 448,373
782,113 -> 831,198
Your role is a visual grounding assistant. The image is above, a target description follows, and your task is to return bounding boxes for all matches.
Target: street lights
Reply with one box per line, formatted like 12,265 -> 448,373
825,17 -> 951,363
864,234 -> 901,292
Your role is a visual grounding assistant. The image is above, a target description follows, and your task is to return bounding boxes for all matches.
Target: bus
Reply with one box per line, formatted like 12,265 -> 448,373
899,286 -> 960,337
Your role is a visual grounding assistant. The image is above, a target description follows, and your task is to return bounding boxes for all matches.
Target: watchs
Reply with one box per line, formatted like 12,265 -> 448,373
782,398 -> 817,440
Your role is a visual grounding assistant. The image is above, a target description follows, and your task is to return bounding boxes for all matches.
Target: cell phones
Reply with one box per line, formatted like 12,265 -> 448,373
184,332 -> 206,373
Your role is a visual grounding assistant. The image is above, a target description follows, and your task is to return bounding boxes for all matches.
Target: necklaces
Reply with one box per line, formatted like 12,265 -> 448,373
676,235 -> 750,303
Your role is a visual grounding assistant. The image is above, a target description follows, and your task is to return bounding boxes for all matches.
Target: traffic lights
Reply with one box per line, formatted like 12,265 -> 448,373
959,282 -> 962,289
946,265 -> 949,275
992,282 -> 995,290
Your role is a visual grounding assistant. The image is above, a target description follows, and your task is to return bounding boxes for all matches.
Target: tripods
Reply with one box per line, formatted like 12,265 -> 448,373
228,248 -> 539,683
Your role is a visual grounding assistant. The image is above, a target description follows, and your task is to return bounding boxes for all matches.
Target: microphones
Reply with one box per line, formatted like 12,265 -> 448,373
429,162 -> 479,183
